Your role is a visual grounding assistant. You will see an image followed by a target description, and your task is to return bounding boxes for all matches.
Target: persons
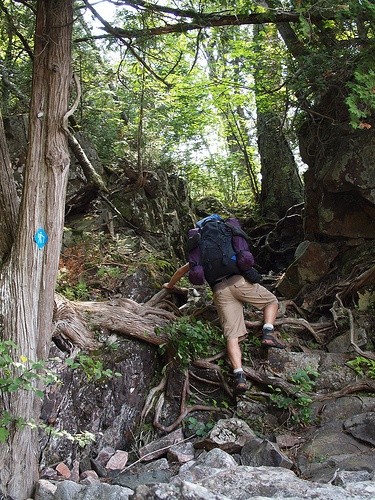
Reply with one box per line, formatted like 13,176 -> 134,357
163,214 -> 288,393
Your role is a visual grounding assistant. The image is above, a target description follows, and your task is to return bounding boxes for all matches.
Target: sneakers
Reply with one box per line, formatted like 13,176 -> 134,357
233,371 -> 252,390
262,327 -> 287,349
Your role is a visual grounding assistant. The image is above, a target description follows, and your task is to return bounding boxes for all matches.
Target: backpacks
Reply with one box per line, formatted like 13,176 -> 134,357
187,215 -> 254,286
